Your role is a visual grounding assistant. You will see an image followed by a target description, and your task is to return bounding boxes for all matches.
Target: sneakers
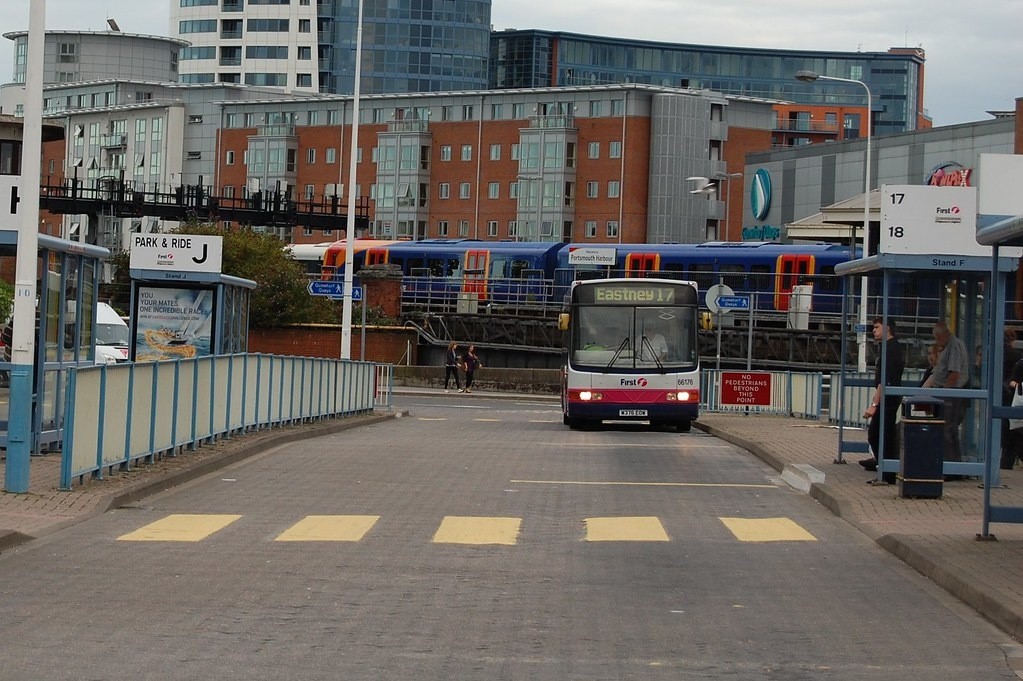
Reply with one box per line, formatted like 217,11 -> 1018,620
458,388 -> 463,392
444,389 -> 449,393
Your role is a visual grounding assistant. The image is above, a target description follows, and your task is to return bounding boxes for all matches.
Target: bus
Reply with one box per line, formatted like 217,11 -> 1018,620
557,277 -> 712,433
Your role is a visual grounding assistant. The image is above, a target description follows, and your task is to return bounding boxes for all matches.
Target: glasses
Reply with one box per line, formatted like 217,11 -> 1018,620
645,328 -> 654,331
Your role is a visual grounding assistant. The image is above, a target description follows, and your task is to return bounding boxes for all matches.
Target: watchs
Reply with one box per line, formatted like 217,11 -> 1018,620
871,402 -> 879,407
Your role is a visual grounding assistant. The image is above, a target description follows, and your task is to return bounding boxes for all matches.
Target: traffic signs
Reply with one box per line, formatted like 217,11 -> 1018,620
713,295 -> 750,311
307,281 -> 343,296
327,287 -> 362,300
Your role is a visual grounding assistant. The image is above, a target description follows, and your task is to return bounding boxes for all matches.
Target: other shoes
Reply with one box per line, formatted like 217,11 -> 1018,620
866,467 -> 877,471
866,478 -> 895,484
466,388 -> 471,393
944,474 -> 969,481
859,458 -> 877,467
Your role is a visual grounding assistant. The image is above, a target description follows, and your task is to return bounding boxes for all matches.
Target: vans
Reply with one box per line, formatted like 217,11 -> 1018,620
63,298 -> 129,366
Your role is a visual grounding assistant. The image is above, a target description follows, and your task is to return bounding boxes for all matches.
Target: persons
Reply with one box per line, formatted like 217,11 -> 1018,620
463,345 -> 482,393
444,341 -> 464,393
635,320 -> 668,362
0,293 -> 41,395
858,315 -> 1023,485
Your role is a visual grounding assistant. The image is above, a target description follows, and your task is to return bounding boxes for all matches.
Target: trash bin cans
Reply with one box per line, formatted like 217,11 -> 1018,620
897,393 -> 947,501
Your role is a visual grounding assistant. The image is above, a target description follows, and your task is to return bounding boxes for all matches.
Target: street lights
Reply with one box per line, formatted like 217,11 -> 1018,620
792,70 -> 872,374
714,171 -> 743,241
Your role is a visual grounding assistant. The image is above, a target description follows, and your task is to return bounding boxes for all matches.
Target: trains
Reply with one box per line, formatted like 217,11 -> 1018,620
319,237 -> 1023,350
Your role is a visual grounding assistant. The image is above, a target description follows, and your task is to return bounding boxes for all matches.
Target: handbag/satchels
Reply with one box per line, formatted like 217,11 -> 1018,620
1009,382 -> 1023,430
476,358 -> 484,368
